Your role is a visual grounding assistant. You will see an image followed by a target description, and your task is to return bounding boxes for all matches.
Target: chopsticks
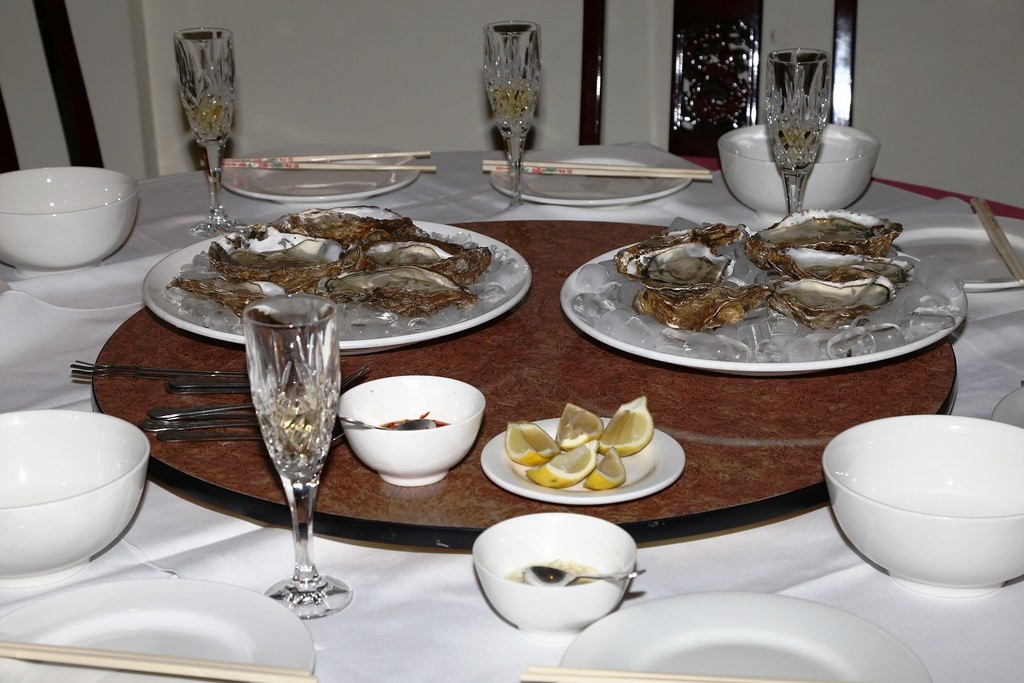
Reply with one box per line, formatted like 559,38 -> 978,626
223,151 -> 437,172
519,665 -> 814,683
482,159 -> 713,181
0,640 -> 317,683
970,197 -> 1024,284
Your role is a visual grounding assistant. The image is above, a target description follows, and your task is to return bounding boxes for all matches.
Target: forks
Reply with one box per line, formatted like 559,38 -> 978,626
165,360 -> 372,394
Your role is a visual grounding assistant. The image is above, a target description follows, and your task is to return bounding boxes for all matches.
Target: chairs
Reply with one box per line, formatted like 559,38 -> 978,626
0,0 -> 106,173
579,0 -> 859,159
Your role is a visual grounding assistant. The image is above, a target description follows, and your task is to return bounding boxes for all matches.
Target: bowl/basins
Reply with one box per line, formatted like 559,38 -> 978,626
340,375 -> 485,487
718,123 -> 880,223
473,512 -> 637,640
0,409 -> 151,590
821,415 -> 1024,598
0,167 -> 140,278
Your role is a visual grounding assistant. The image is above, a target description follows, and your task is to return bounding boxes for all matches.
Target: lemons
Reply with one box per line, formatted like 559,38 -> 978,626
584,446 -> 627,490
504,420 -> 561,465
597,394 -> 654,457
525,439 -> 600,488
555,402 -> 605,450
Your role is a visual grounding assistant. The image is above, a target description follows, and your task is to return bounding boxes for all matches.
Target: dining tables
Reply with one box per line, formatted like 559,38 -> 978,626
0,139 -> 1024,683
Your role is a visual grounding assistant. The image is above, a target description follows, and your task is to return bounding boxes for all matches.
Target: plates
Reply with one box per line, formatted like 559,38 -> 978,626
0,579 -> 316,683
480,418 -> 686,504
143,218 -> 532,356
887,214 -> 1024,293
992,385 -> 1024,429
560,232 -> 967,376
560,592 -> 934,683
221,146 -> 420,208
489,145 -> 693,209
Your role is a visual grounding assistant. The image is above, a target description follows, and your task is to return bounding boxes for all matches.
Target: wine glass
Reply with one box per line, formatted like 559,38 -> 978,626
243,295 -> 352,619
173,27 -> 249,237
765,47 -> 831,213
483,20 -> 542,218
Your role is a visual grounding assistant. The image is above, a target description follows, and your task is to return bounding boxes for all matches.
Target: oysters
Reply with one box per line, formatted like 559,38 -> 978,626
169,205 -> 493,317
612,207 -> 914,334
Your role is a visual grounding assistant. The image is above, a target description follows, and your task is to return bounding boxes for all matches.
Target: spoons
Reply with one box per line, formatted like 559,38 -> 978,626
340,417 -> 435,431
523,566 -> 646,590
